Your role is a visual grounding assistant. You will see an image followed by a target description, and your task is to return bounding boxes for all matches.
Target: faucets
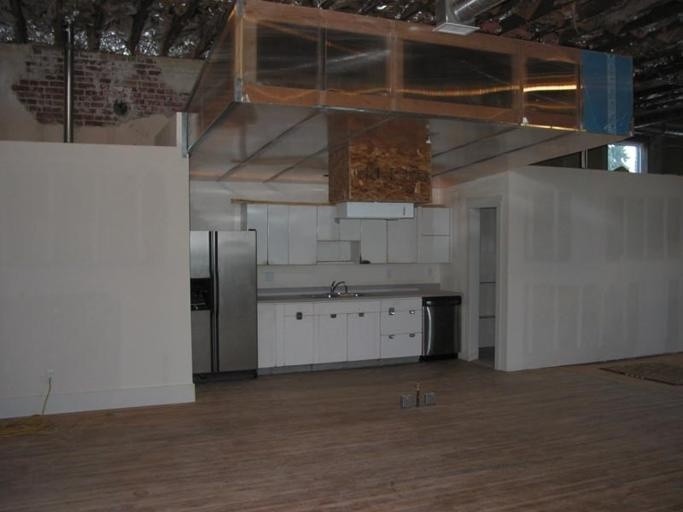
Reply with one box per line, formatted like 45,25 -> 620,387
330,280 -> 346,292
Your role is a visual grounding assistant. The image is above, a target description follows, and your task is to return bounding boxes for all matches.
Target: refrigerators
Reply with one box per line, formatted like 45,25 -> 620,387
190,228 -> 258,385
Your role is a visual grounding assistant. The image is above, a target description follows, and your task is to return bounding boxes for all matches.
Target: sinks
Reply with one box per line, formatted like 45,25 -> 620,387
333,292 -> 362,296
302,293 -> 334,298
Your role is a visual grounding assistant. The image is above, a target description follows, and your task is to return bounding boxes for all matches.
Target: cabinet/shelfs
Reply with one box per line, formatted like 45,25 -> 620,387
257,300 -> 423,369
241,204 -> 452,264
